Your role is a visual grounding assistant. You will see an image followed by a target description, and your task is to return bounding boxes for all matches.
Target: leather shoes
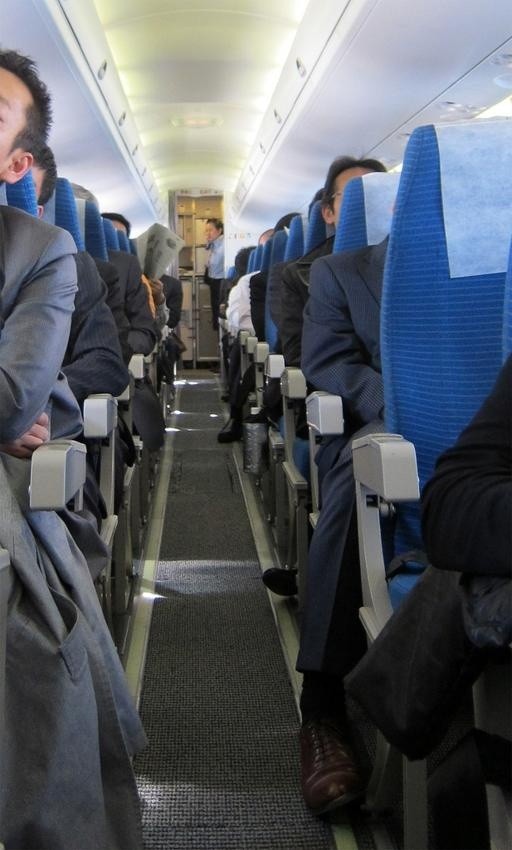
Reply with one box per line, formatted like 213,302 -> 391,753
300,715 -> 367,817
263,569 -> 299,597
217,419 -> 243,443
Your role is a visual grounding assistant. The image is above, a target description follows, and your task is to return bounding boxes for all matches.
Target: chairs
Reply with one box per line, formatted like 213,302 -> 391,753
0,119 -> 208,634
216,119 -> 509,843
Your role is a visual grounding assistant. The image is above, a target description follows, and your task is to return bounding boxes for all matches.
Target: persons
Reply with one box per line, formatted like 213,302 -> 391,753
0,48 -> 182,849
204,217 -> 225,373
216,153 -> 512,848
